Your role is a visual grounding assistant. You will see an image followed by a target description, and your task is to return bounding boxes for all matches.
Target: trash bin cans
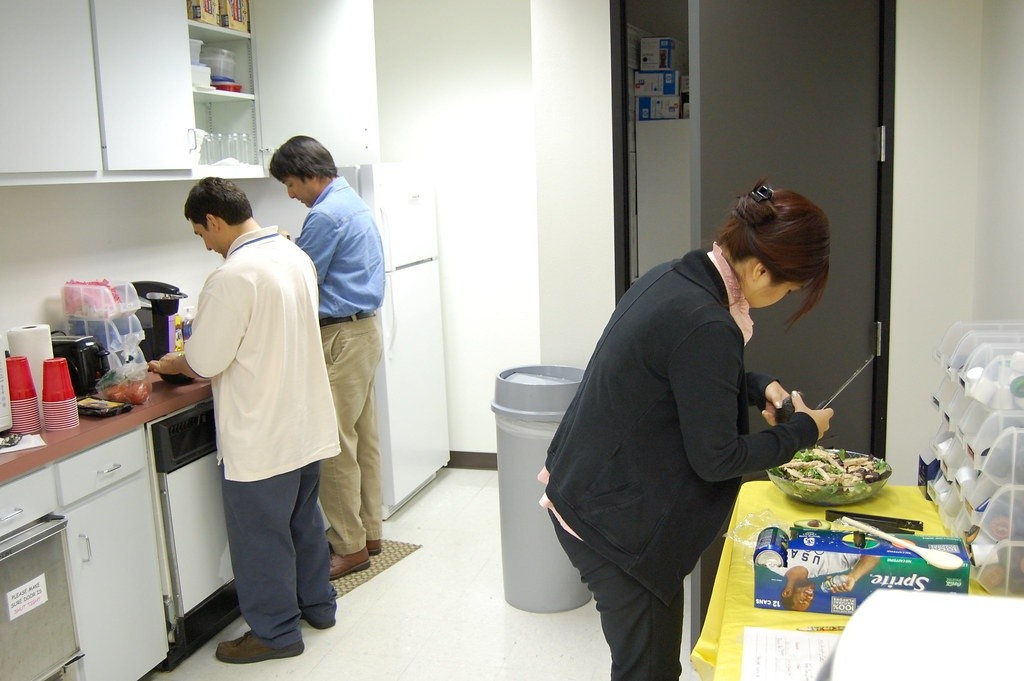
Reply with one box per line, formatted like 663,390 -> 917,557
492,367 -> 594,614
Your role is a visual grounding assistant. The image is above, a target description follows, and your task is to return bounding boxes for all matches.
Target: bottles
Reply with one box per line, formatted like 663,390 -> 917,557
175,314 -> 184,351
820,575 -> 849,593
183,308 -> 192,342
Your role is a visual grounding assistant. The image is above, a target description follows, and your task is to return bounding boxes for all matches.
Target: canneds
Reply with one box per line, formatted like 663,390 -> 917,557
753,526 -> 790,569
821,575 -> 847,593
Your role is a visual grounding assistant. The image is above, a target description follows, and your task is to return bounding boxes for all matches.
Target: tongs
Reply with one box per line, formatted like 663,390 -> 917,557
826,510 -> 923,535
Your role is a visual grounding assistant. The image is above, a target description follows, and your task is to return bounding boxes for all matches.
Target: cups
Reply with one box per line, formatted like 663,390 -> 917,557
43,358 -> 79,430
200,133 -> 251,167
4,357 -> 41,434
189,39 -> 204,64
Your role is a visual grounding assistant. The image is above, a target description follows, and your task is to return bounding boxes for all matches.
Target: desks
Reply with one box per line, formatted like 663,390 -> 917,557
710,483 -> 1024,681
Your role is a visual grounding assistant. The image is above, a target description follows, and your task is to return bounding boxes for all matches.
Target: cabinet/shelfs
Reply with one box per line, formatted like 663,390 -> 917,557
0,371 -> 212,681
0,0 -> 272,187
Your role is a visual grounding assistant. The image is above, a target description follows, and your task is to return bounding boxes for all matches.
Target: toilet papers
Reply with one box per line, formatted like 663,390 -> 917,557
6,323 -> 55,421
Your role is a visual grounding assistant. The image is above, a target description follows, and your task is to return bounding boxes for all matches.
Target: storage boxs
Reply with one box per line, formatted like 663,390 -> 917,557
917,320 -> 1024,597
107,346 -> 149,373
219,0 -> 250,33
683,103 -> 690,119
636,96 -> 684,120
62,280 -> 142,320
191,65 -> 212,86
641,36 -> 689,71
635,69 -> 681,96
186,0 -> 219,26
69,314 -> 145,351
753,531 -> 972,617
681,75 -> 689,93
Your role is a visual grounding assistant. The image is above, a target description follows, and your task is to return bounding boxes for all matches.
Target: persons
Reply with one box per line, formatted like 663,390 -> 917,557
267,135 -> 388,581
537,174 -> 834,681
765,548 -> 881,613
150,177 -> 344,664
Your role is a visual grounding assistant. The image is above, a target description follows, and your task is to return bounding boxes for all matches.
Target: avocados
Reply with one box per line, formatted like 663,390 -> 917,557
794,518 -> 831,531
776,391 -> 806,425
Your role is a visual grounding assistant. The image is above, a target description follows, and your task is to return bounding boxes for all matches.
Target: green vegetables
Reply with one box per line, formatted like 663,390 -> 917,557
767,445 -> 888,505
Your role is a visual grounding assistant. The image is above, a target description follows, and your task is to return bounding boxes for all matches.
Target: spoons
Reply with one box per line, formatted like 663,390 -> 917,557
0,435 -> 22,449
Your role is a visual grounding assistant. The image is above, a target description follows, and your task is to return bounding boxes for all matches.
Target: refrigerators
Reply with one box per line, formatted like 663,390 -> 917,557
336,163 -> 450,522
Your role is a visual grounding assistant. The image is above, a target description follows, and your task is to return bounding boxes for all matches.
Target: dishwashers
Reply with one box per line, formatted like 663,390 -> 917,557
151,401 -> 239,648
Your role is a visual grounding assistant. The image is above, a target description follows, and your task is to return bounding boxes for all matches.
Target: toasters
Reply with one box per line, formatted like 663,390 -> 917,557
51,335 -> 110,396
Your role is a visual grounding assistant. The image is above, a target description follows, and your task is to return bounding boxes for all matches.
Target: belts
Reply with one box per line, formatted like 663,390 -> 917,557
319,310 -> 376,328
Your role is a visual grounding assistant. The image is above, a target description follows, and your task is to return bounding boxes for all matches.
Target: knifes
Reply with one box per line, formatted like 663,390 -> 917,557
814,355 -> 874,411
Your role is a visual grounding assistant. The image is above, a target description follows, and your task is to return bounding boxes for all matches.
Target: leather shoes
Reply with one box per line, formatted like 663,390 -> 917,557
329,546 -> 371,580
365,539 -> 381,556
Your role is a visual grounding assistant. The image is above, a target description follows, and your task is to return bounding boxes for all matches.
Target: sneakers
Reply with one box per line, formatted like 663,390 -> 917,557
300,615 -> 335,629
215,630 -> 304,664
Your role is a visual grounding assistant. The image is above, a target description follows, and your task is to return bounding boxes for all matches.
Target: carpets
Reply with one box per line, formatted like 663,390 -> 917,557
330,539 -> 424,598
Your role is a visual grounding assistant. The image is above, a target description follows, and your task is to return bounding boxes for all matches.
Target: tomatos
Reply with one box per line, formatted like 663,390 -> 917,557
107,380 -> 152,406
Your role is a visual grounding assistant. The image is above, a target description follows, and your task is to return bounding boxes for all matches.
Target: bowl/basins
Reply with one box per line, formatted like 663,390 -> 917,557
210,83 -> 242,93
766,449 -> 892,506
